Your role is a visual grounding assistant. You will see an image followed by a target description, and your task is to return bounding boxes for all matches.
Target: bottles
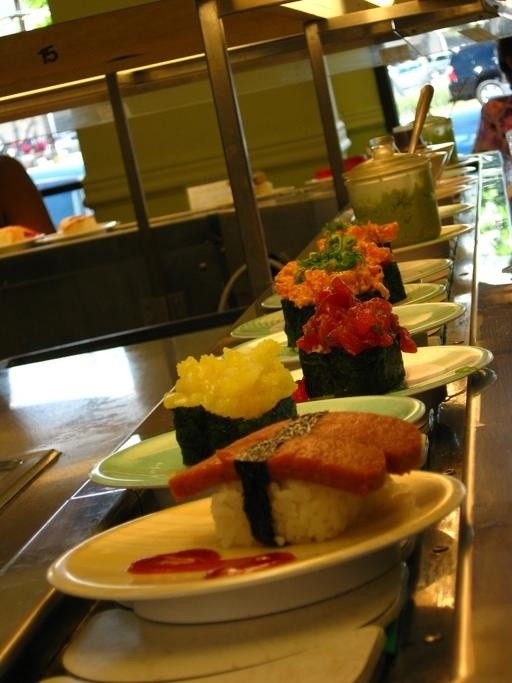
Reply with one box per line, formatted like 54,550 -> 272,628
342,135 -> 442,250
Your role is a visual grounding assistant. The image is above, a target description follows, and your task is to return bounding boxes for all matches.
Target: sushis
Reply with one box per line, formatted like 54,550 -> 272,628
164,215 -> 422,547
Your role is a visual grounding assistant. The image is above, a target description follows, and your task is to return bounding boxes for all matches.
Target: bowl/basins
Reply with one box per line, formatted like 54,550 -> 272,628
399,141 -> 455,182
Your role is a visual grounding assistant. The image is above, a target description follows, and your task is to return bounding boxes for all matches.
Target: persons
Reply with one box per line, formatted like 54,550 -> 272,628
0,155 -> 56,234
471,37 -> 512,158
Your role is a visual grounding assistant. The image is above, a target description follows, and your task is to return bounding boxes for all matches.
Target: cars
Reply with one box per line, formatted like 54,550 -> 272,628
391,39 -> 512,104
55,131 -> 81,154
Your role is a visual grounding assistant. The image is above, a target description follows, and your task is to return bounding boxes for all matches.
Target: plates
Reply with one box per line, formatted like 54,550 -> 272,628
290,345 -> 493,396
86,394 -> 429,491
390,223 -> 475,252
260,258 -> 455,309
207,302 -> 468,363
44,468 -> 466,626
